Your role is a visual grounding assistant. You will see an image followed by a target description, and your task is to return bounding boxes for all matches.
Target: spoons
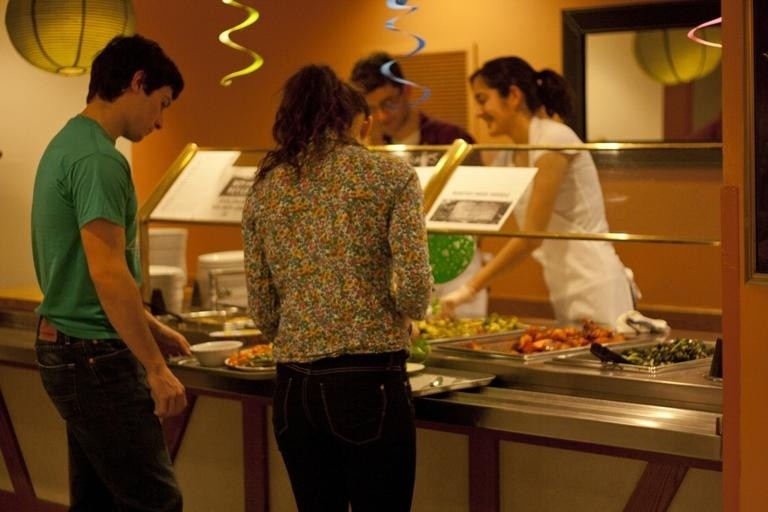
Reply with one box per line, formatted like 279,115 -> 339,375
420,375 -> 443,390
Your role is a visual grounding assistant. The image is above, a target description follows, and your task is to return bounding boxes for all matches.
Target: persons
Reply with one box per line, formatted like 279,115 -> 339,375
435,53 -> 644,332
240,62 -> 431,511
348,50 -> 481,168
31,32 -> 197,510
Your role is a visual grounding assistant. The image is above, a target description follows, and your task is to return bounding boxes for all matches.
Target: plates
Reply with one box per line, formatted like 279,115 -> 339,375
224,347 -> 277,371
211,329 -> 261,338
196,249 -> 249,309
149,264 -> 186,314
406,362 -> 426,374
148,228 -> 189,266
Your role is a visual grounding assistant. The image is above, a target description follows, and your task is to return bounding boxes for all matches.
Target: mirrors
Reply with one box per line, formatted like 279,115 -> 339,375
562,0 -> 722,169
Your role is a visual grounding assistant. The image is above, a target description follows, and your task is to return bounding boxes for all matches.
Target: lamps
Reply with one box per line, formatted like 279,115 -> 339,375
5,0 -> 135,78
633,26 -> 722,88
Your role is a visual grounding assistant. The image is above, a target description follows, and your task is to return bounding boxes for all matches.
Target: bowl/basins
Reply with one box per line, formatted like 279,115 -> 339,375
189,340 -> 244,367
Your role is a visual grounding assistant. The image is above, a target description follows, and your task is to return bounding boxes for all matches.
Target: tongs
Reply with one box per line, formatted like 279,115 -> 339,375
588,342 -> 630,365
